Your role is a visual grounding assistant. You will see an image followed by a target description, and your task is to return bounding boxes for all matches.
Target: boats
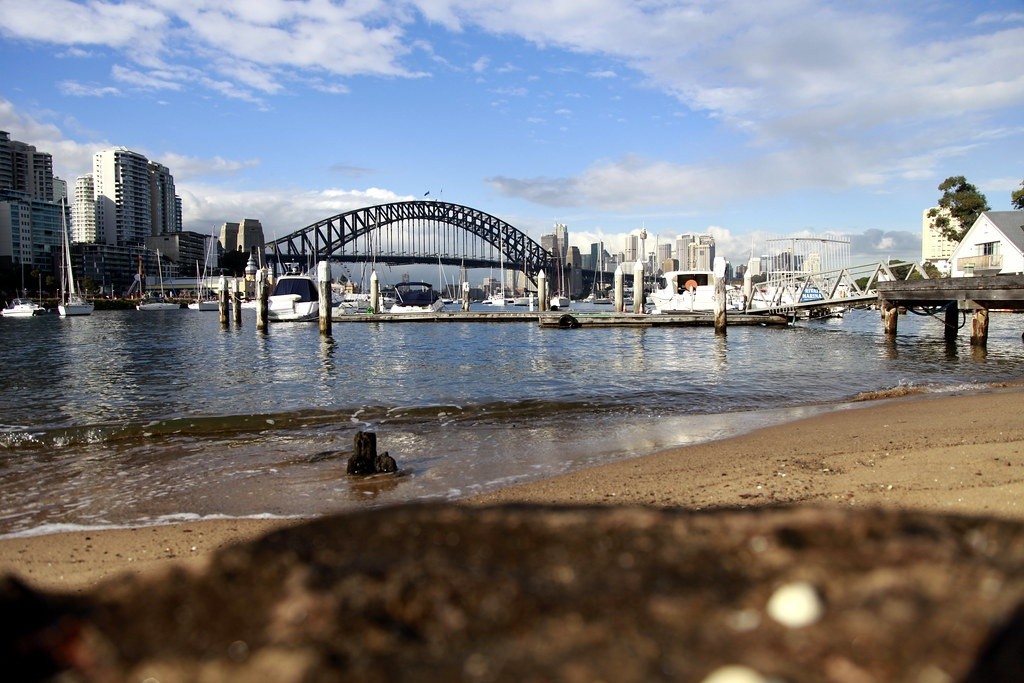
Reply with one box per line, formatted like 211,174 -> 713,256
0,288 -> 46,317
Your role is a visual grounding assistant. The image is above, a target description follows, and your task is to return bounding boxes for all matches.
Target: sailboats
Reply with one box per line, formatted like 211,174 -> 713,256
135,247 -> 182,311
186,218 -> 849,324
57,196 -> 95,316
